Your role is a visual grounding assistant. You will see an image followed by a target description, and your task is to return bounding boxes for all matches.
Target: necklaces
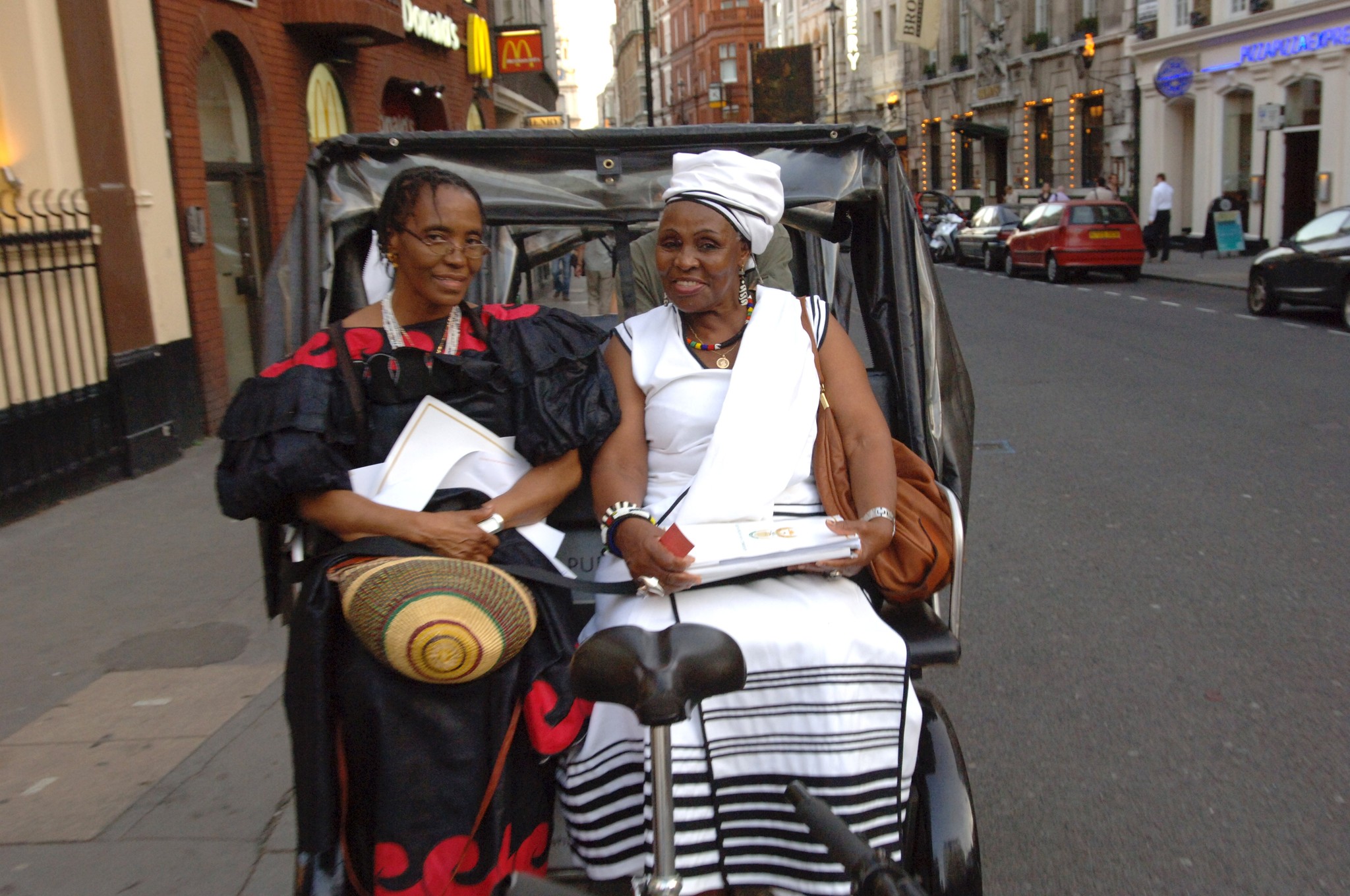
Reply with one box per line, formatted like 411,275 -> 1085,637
683,291 -> 757,352
395,311 -> 454,354
684,316 -> 742,369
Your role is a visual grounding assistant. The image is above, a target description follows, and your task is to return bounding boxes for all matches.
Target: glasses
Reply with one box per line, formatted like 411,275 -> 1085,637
398,223 -> 492,259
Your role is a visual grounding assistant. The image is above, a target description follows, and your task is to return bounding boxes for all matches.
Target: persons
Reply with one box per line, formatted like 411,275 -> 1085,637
215,146 -> 623,896
994,170 -> 1123,205
555,134 -> 932,894
549,249 -> 572,303
1141,172 -> 1175,264
575,234 -> 619,316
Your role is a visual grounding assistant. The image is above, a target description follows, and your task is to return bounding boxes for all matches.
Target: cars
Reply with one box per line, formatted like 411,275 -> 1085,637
1243,204 -> 1350,329
917,189 -> 1147,284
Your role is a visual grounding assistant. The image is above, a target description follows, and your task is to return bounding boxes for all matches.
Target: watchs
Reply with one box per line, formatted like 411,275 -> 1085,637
859,504 -> 897,544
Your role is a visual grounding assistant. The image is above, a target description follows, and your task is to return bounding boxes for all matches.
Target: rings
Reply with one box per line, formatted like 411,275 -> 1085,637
634,572 -> 665,600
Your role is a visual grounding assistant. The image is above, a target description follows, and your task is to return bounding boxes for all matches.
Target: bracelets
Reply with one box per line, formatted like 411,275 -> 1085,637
577,262 -> 583,267
599,497 -> 655,559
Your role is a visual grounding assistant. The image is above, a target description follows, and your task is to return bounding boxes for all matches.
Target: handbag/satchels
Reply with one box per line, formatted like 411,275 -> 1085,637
331,557 -> 542,686
800,294 -> 955,603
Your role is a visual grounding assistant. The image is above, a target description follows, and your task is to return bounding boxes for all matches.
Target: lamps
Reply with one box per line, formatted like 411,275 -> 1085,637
1250,1 -> 1271,13
399,79 -> 427,96
2,164 -> 24,189
1250,174 -> 1263,204
1316,172 -> 1332,204
887,94 -> 900,112
1135,22 -> 1153,42
1189,12 -> 1209,28
422,83 -> 446,99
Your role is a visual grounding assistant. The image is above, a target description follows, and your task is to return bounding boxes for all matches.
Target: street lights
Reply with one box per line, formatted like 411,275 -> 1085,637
825,1 -> 844,119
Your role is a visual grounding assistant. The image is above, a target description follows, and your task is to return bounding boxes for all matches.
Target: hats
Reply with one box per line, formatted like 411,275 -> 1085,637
659,150 -> 785,254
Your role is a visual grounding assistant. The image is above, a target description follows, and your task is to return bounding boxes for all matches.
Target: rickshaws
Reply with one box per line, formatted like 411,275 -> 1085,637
251,120 -> 980,896
